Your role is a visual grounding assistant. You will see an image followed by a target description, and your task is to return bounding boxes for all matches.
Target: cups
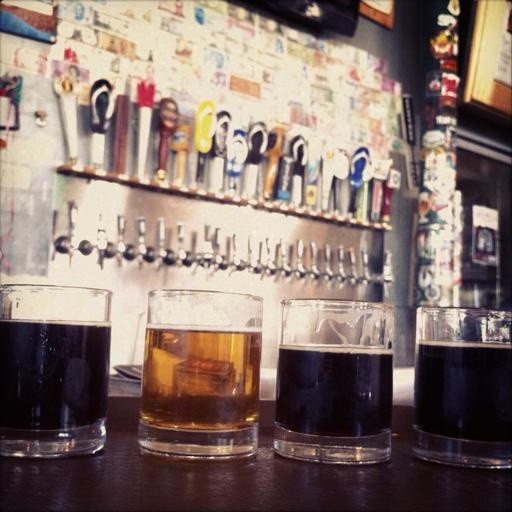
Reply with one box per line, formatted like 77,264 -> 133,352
0,283 -> 112,457
135,289 -> 264,460
409,306 -> 512,469
273,297 -> 396,465
